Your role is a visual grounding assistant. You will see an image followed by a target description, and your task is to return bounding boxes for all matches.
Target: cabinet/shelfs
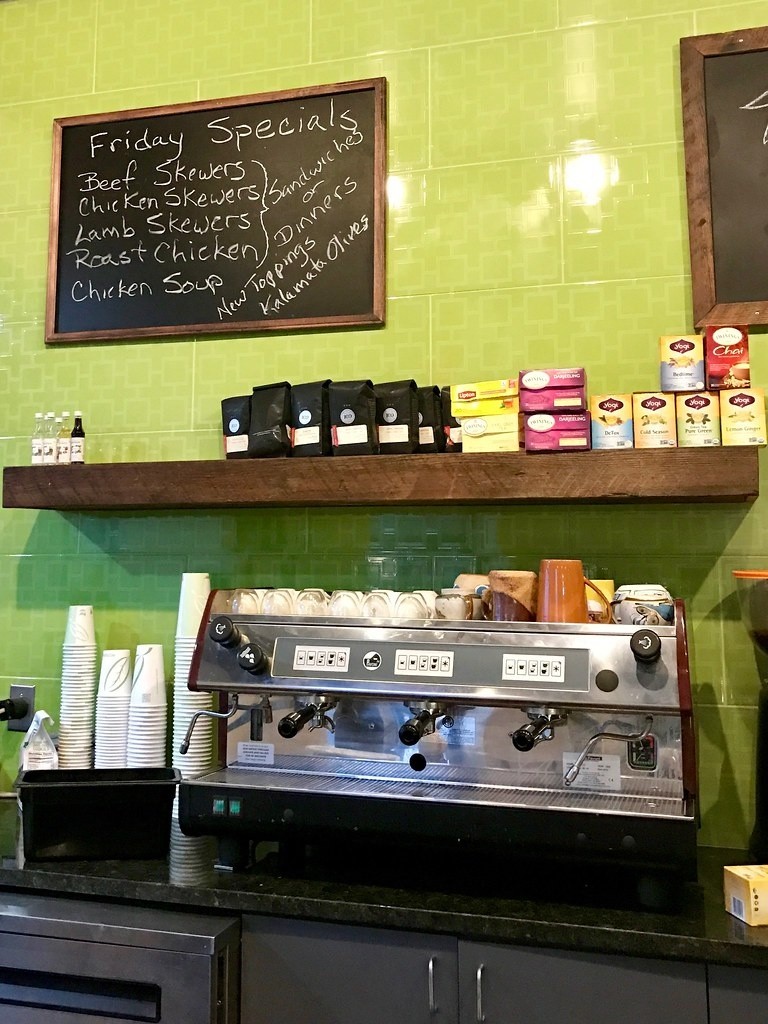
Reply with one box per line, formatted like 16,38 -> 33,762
237,913 -> 768,1024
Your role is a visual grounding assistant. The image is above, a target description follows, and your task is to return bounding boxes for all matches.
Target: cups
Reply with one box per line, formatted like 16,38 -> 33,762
229,588 -> 483,620
490,571 -> 536,621
58,572 -> 219,889
582,578 -> 614,614
537,558 -> 613,624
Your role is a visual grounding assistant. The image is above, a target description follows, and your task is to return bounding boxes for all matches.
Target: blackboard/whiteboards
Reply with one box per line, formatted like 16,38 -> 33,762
44,76 -> 390,347
678,24 -> 768,336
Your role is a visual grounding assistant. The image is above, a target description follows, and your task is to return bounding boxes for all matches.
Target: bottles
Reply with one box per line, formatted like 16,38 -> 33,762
29,412 -> 85,464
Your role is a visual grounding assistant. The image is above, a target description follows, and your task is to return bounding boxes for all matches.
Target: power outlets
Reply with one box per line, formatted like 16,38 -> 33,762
7,684 -> 36,732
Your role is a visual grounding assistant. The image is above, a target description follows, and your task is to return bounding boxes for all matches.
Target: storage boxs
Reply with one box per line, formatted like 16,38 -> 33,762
451,323 -> 767,454
14,766 -> 182,863
723,864 -> 768,926
